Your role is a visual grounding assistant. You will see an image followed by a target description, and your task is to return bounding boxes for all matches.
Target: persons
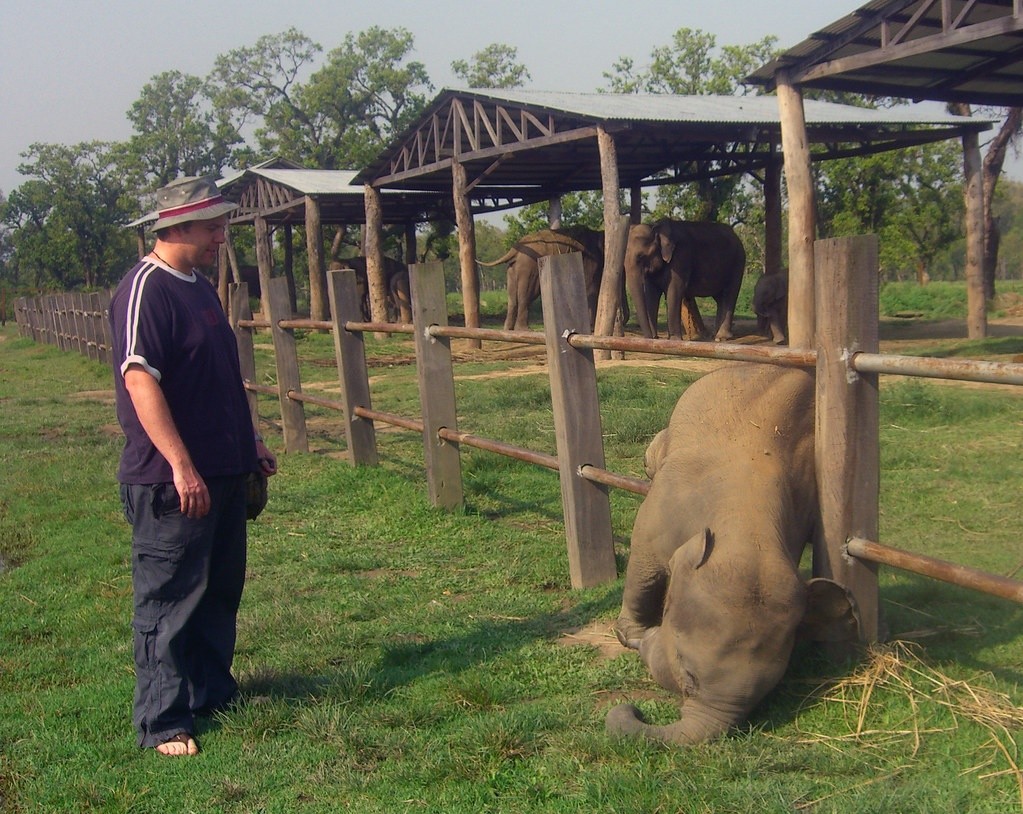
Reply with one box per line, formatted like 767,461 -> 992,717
105,175 -> 278,760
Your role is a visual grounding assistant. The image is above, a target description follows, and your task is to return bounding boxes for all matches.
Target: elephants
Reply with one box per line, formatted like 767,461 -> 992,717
328,256 -> 413,324
603,362 -> 861,752
475,216 -> 789,345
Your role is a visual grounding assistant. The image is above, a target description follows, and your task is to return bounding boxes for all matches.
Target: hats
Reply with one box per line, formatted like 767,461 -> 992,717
148,176 -> 240,233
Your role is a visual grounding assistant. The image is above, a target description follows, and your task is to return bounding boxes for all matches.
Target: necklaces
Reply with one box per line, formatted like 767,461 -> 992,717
152,249 -> 192,276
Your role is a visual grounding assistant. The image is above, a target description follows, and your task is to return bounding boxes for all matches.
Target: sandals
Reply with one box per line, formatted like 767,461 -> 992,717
164,733 -> 201,755
233,691 -> 270,713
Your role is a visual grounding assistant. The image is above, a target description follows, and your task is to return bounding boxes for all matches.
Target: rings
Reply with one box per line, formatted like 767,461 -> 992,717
258,457 -> 266,463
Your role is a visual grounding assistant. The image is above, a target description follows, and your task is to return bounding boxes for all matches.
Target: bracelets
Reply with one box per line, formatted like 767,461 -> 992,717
255,435 -> 263,442
